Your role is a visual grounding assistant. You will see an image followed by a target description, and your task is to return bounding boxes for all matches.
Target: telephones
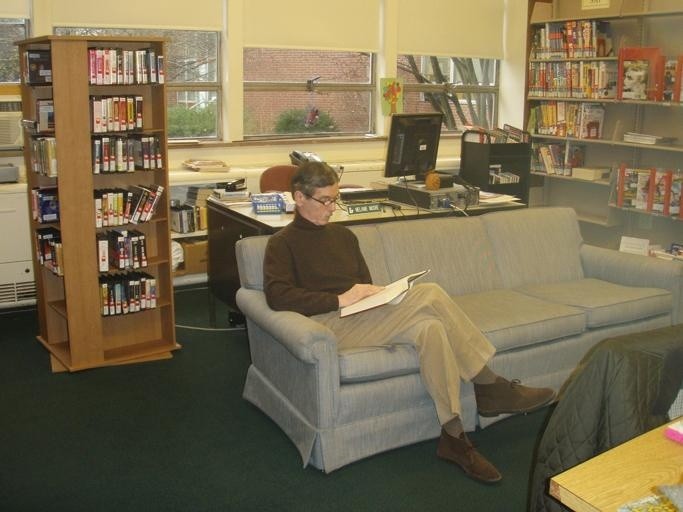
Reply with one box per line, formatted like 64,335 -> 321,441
288,150 -> 322,165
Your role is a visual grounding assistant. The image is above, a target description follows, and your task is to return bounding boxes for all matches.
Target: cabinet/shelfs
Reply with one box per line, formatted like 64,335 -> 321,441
527,10 -> 682,260
168,175 -> 250,287
12,36 -> 184,374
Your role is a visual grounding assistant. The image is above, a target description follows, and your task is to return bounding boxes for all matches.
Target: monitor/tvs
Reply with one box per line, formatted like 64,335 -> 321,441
383,111 -> 444,178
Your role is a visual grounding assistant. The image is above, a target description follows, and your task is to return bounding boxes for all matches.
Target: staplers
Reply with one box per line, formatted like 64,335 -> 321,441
224,178 -> 247,192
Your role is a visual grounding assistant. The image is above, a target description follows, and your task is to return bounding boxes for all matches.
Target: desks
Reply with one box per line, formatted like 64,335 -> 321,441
547,414 -> 683,512
205,189 -> 527,328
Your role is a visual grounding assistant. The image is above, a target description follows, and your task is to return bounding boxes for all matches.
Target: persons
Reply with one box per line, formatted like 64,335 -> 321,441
265,161 -> 558,482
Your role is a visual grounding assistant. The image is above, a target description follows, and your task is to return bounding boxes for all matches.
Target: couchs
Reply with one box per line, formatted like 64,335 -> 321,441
234,206 -> 683,476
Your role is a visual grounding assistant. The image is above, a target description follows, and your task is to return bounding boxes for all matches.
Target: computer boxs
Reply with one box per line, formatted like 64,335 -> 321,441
390,180 -> 480,210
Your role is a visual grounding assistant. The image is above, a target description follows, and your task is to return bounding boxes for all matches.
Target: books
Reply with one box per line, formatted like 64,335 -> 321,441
341,269 -> 432,317
22,47 -> 63,277
97,229 -> 147,272
94,184 -> 164,228
526,18 -> 683,261
187,159 -> 229,172
487,163 -> 520,184
168,186 -> 250,234
91,135 -> 162,173
90,96 -> 144,133
90,46 -> 165,85
99,270 -> 156,316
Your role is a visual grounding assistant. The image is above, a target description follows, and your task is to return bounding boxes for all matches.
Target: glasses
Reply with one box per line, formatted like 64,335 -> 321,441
297,190 -> 340,205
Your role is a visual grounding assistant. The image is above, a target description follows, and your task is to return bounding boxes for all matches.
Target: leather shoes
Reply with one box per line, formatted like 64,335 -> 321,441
435,427 -> 504,485
474,375 -> 557,418
